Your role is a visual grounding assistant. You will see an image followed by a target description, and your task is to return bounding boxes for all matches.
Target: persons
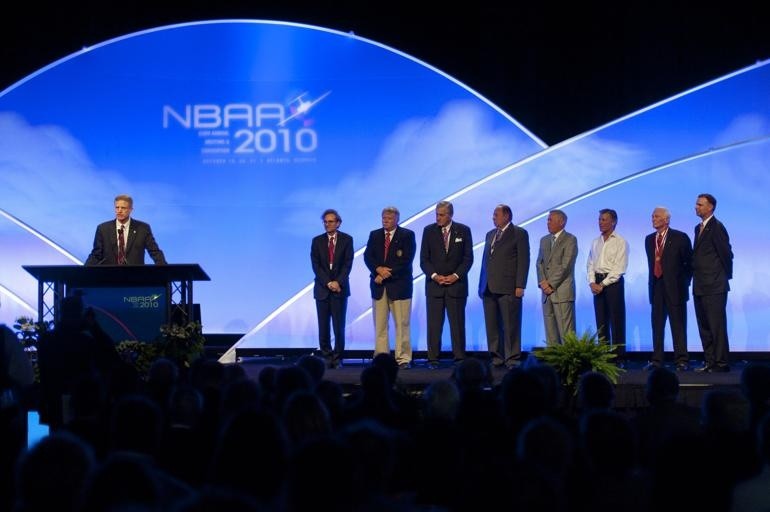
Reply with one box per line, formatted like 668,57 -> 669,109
536,209 -> 579,349
643,207 -> 693,373
477,203 -> 530,369
83,193 -> 168,267
586,209 -> 630,372
311,208 -> 354,363
691,192 -> 733,374
1,296 -> 769,511
363,208 -> 417,371
419,200 -> 475,369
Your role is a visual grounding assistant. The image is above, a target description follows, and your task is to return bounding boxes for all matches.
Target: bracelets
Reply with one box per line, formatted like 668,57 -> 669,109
600,282 -> 605,288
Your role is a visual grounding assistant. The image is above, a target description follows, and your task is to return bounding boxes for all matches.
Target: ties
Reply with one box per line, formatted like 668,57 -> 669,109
698,223 -> 704,235
118,226 -> 125,264
654,233 -> 662,277
329,237 -> 334,264
551,236 -> 555,246
493,230 -> 500,247
384,233 -> 390,259
443,228 -> 448,252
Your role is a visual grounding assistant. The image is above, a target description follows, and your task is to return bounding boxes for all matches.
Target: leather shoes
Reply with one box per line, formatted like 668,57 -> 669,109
429,365 -> 438,369
642,363 -> 729,373
399,363 -> 411,369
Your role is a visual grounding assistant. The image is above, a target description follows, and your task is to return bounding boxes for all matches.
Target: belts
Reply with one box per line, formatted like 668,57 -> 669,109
595,273 -> 608,277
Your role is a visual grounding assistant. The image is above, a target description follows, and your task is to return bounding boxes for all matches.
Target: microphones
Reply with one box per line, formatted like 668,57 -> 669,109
96,258 -> 107,265
121,256 -> 128,264
